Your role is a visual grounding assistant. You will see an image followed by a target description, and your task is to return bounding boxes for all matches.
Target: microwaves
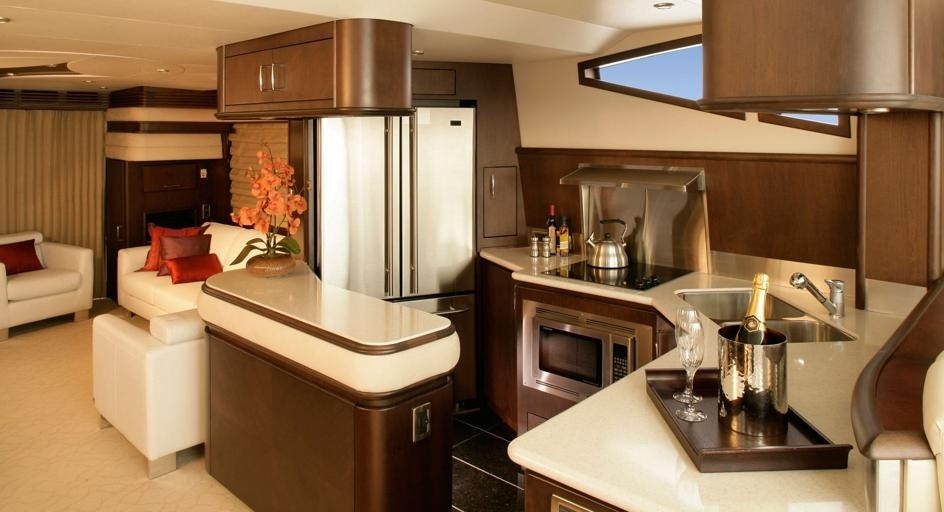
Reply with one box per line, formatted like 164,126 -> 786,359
513,287 -> 653,404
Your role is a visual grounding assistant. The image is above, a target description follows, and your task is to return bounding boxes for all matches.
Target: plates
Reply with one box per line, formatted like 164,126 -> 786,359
644,367 -> 853,474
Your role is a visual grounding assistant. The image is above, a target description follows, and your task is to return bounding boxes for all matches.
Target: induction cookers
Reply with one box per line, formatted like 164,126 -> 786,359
541,259 -> 694,291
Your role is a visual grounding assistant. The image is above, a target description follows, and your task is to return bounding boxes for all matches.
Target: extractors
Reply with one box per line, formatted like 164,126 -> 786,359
559,164 -> 701,193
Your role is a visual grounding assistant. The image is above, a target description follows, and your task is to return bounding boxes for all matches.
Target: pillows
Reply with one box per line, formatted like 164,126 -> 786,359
0,238 -> 45,276
133,222 -> 223,284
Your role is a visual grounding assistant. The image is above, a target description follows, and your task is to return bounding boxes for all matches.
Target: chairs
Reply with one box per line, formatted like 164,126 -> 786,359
0,232 -> 94,342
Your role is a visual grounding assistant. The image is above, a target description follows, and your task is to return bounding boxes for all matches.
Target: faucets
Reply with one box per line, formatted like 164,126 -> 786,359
790,272 -> 846,320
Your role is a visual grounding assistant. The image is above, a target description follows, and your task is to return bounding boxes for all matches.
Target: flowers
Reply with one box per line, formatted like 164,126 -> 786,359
229,141 -> 308,265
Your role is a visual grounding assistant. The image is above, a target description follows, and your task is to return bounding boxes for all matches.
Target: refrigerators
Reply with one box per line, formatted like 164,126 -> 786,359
314,110 -> 478,402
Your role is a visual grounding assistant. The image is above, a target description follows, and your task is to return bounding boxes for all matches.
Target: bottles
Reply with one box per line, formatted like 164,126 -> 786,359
734,271 -> 770,344
529,203 -> 570,257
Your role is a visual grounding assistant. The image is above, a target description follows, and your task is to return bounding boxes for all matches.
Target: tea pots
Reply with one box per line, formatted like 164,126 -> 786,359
585,217 -> 629,269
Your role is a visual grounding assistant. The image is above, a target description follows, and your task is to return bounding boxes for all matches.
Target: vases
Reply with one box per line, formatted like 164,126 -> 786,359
246,236 -> 296,278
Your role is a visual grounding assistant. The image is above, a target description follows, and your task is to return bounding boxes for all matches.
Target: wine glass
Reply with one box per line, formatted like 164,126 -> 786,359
672,308 -> 702,404
675,321 -> 706,422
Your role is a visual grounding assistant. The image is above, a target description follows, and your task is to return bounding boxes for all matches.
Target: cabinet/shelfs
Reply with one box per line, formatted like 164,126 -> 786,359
214,18 -> 417,120
104,157 -> 212,307
697,1 -> 943,113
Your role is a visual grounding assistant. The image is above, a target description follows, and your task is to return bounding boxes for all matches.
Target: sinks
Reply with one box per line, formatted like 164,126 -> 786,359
723,315 -> 857,346
675,286 -> 806,326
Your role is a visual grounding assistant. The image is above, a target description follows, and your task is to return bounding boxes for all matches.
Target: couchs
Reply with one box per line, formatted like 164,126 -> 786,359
91,309 -> 206,480
118,222 -> 290,319
